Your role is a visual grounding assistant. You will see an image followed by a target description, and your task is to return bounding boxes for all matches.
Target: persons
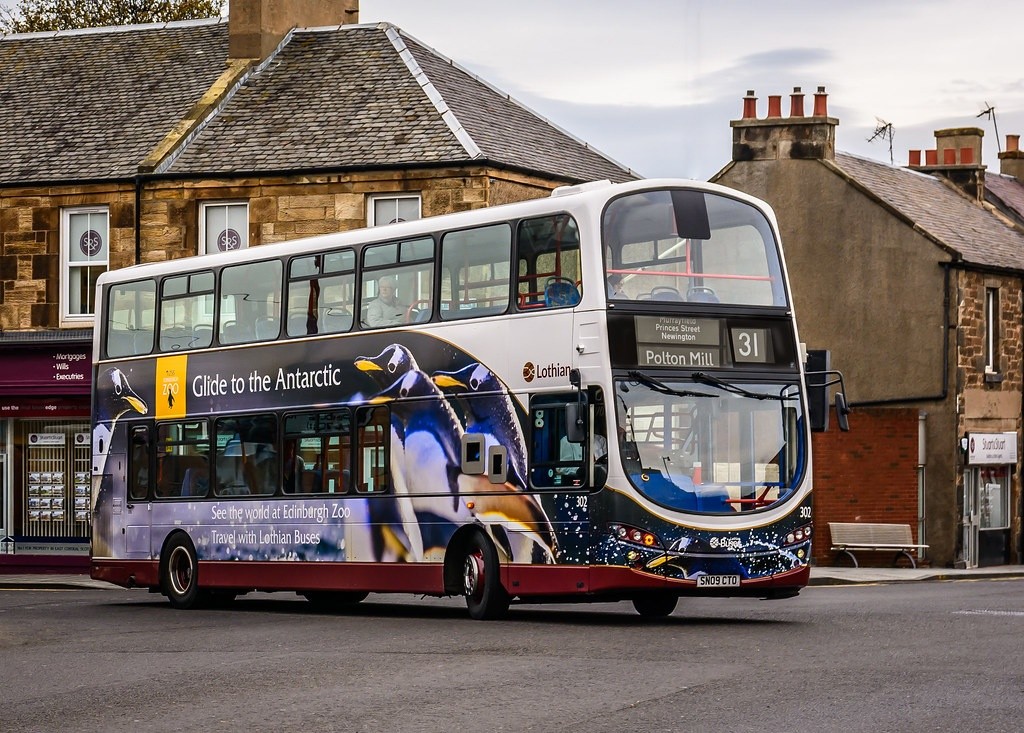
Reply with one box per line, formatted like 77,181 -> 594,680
368,275 -> 415,330
554,433 -> 608,482
270,433 -> 306,494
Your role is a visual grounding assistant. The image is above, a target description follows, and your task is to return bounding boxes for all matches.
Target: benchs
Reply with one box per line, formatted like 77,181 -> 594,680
827,522 -> 930,568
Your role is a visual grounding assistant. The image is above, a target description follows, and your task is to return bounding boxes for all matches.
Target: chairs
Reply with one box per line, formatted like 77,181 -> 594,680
133,445 -> 351,498
111,275 -> 721,358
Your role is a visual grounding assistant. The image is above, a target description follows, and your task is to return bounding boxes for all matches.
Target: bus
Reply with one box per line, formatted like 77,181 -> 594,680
89,178 -> 851,626
